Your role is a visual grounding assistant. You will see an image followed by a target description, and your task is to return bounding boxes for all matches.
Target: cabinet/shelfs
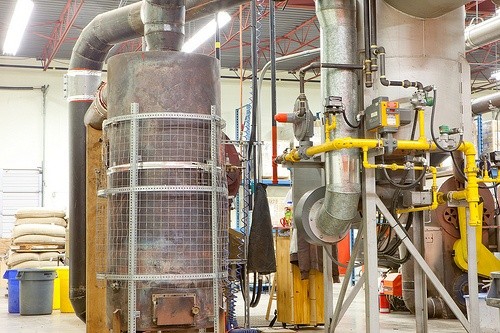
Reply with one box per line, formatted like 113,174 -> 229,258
0,83 -> 49,238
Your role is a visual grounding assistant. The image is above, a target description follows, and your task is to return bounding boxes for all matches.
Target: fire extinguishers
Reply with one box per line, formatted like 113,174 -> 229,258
378,271 -> 391,316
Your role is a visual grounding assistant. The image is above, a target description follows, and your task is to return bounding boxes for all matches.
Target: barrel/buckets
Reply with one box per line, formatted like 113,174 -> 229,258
41,262 -> 81,313
2,268 -> 40,314
16,266 -> 58,315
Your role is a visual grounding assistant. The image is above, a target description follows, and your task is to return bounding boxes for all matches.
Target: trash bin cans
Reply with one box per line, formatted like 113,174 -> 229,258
2,265 -> 74,318
